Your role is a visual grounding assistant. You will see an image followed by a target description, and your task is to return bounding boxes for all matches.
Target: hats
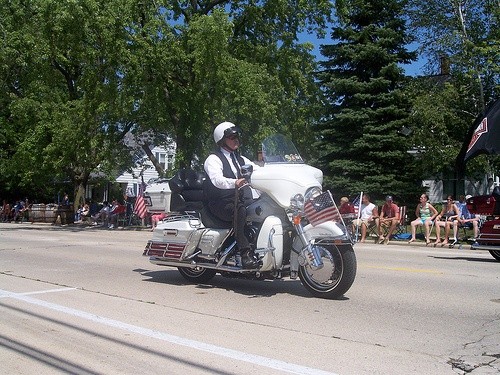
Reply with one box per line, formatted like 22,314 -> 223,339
386,196 -> 392,200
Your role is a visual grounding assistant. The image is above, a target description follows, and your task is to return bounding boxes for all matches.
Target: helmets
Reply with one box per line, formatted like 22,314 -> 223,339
213,122 -> 242,143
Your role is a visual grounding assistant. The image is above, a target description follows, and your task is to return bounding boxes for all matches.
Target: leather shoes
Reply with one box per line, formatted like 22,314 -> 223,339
242,252 -> 257,268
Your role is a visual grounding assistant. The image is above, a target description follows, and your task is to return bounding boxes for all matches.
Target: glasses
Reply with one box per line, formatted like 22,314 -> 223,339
224,133 -> 239,140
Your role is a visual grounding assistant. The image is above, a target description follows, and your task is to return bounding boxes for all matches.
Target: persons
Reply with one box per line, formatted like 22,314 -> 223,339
484,178 -> 493,195
0,197 -> 38,223
203,122 -> 263,269
434,195 -> 459,245
74,196 -> 131,228
61,191 -> 70,206
339,197 -> 355,226
375,196 -> 400,245
150,213 -> 168,232
449,195 -> 481,246
409,194 -> 438,244
351,194 -> 378,244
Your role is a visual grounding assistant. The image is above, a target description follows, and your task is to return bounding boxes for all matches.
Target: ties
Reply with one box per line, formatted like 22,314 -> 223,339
230,153 -> 240,175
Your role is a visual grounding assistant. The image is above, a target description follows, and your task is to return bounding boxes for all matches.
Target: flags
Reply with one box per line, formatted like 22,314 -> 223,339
304,190 -> 340,227
133,194 -> 148,218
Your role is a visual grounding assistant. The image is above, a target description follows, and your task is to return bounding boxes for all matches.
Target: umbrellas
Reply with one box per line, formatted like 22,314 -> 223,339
454,97 -> 500,164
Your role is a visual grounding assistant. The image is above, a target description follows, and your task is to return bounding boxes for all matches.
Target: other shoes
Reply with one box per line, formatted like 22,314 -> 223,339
383,238 -> 389,244
377,238 -> 384,244
450,240 -> 457,246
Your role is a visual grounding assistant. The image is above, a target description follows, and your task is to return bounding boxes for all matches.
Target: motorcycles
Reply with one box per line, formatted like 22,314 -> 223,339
465,186 -> 500,264
142,131 -> 358,299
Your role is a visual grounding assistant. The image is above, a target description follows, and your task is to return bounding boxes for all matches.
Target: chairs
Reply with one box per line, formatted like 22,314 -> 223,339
417,202 -> 477,238
340,205 -> 408,240
